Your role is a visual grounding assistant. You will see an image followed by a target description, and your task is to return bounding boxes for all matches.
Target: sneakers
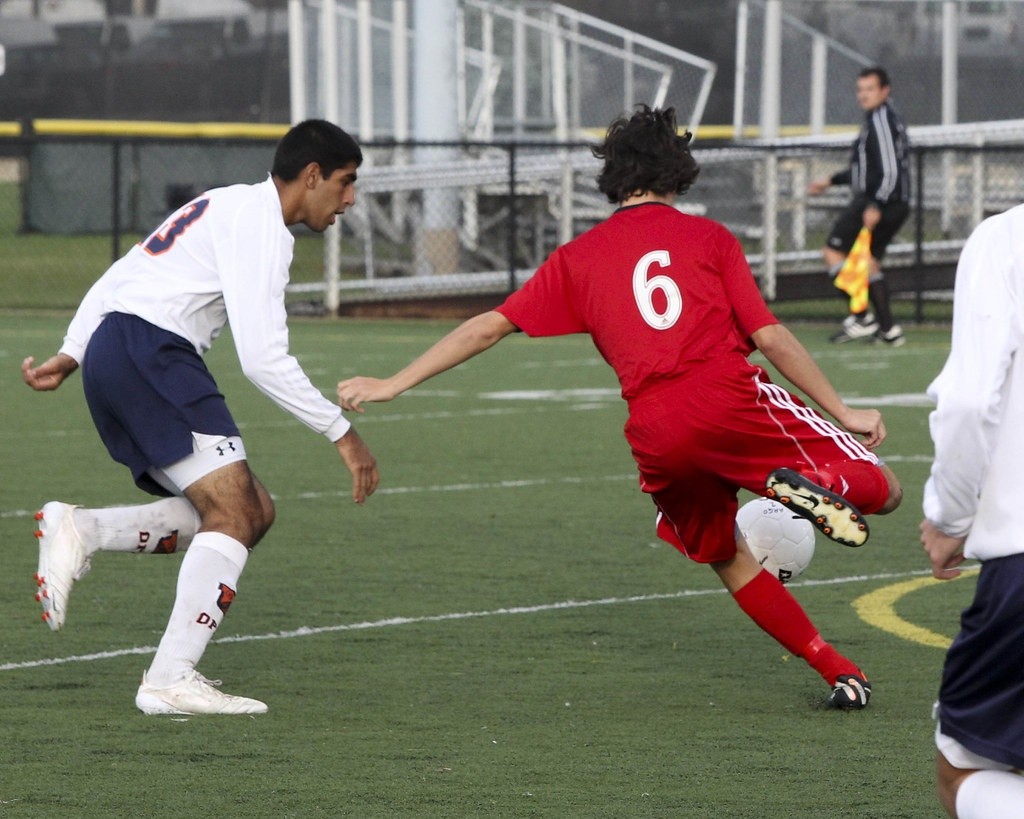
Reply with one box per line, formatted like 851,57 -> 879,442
828,672 -> 872,707
829,311 -> 880,344
34,500 -> 93,631
766,467 -> 870,547
874,322 -> 906,347
136,665 -> 268,715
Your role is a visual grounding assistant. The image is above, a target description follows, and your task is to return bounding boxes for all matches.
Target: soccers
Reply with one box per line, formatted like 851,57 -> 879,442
734,496 -> 815,583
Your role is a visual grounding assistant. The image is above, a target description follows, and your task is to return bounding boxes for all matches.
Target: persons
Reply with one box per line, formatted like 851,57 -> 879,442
336,102 -> 904,710
920,198 -> 1023,819
807,66 -> 911,347
21,119 -> 380,716
